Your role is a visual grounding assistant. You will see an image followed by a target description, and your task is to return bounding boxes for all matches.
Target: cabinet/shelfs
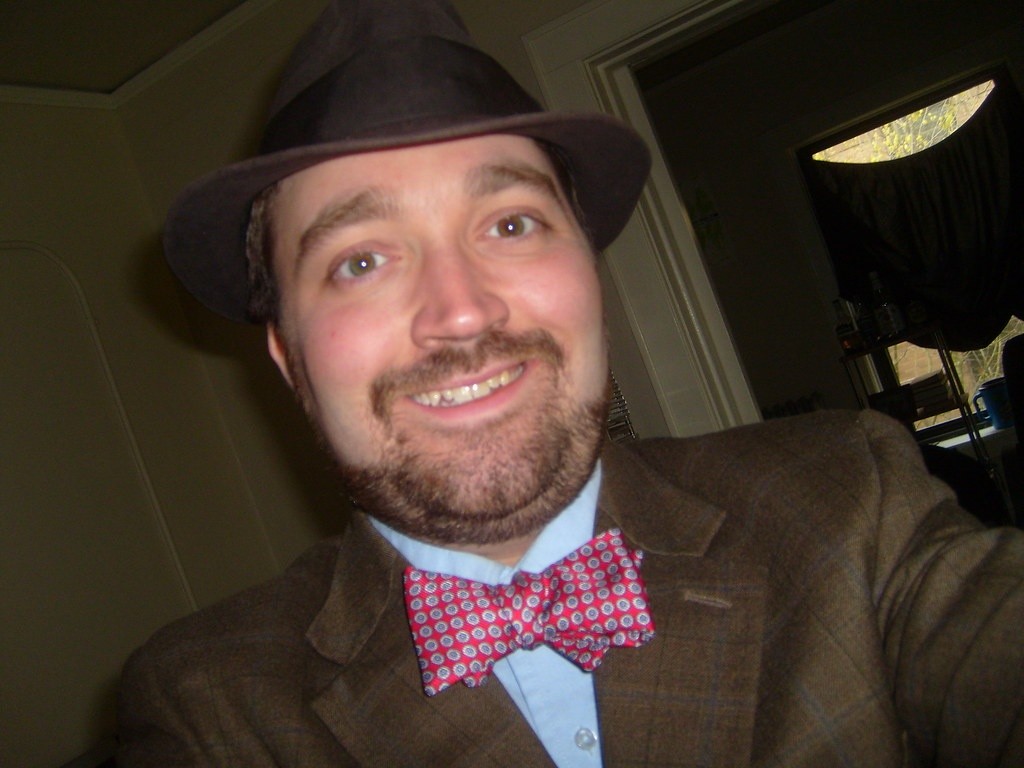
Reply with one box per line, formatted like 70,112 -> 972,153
839,318 -> 1016,507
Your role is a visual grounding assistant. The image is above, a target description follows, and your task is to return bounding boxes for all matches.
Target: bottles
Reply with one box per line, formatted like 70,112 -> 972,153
831,286 -> 929,359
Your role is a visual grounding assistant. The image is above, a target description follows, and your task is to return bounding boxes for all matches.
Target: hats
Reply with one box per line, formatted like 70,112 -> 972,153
164,1 -> 656,322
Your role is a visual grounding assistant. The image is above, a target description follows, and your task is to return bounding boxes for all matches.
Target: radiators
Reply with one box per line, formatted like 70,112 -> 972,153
757,393 -> 824,419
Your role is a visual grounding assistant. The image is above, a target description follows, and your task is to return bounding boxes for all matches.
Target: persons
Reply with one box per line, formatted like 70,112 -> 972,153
110,37 -> 1024,768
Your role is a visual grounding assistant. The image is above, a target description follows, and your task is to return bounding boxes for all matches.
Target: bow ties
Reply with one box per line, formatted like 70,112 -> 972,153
402,528 -> 658,696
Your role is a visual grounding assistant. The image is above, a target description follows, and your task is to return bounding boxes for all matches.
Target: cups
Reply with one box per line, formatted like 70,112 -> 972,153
973,376 -> 1014,430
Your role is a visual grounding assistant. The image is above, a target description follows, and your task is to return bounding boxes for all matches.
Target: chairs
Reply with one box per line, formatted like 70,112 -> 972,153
871,375 -> 986,471
